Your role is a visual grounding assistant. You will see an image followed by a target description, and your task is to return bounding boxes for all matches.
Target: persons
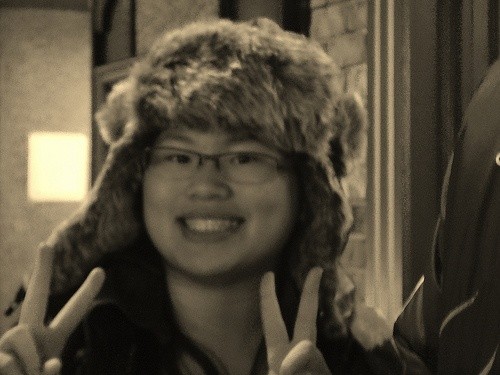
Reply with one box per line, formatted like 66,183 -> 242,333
0,18 -> 429,375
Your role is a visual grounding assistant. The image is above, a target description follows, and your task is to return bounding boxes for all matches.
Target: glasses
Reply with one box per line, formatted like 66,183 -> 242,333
140,141 -> 296,186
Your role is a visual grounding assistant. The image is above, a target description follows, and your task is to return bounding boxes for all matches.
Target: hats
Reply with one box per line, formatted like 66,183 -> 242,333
25,17 -> 367,349
